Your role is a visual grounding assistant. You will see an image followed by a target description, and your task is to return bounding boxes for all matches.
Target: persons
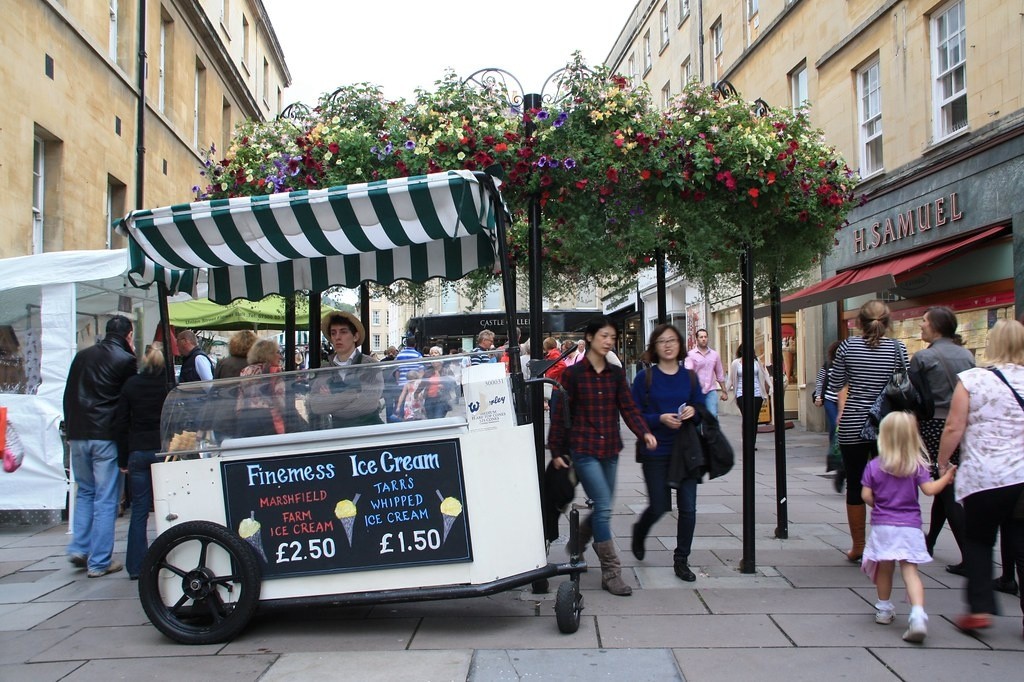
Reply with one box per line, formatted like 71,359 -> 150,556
117,340 -> 173,581
174,329 -> 317,442
63,314 -> 138,578
628,325 -> 707,581
548,315 -> 658,595
937,320 -> 1024,630
722,342 -> 774,451
830,300 -> 911,560
308,309 -> 385,427
687,329 -> 728,421
904,306 -> 975,574
858,410 -> 956,642
380,325 -> 622,424
813,341 -> 849,490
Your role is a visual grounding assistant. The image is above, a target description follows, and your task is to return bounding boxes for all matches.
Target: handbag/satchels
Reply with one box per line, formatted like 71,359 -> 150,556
887,338 -> 921,411
812,362 -> 829,405
825,426 -> 843,472
3,420 -> 24,472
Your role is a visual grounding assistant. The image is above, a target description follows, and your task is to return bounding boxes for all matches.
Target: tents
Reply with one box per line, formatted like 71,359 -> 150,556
134,290 -> 340,330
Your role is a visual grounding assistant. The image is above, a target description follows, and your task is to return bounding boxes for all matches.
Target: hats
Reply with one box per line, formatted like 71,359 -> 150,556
322,310 -> 365,347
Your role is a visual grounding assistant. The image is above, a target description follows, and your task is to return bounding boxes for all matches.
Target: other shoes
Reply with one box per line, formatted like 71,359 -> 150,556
632,522 -> 645,560
875,610 -> 896,623
71,552 -> 87,567
673,564 -> 696,581
945,562 -> 966,576
958,614 -> 992,629
834,475 -> 844,493
991,575 -> 1018,594
902,621 -> 926,641
87,560 -> 123,576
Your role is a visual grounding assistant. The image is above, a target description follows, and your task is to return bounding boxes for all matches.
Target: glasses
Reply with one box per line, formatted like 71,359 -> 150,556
654,338 -> 677,346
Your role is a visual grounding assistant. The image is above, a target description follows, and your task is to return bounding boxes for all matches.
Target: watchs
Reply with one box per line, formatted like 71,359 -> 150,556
935,462 -> 949,469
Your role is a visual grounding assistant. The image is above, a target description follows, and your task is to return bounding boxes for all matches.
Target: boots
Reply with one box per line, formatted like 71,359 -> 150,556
845,502 -> 866,560
592,538 -> 633,594
564,517 -> 593,562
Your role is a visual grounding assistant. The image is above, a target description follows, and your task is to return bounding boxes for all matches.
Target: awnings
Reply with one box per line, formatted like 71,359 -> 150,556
749,225 -> 1005,319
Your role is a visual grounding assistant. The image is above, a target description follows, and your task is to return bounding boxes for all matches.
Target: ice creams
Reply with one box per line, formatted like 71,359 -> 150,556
781,336 -> 795,385
